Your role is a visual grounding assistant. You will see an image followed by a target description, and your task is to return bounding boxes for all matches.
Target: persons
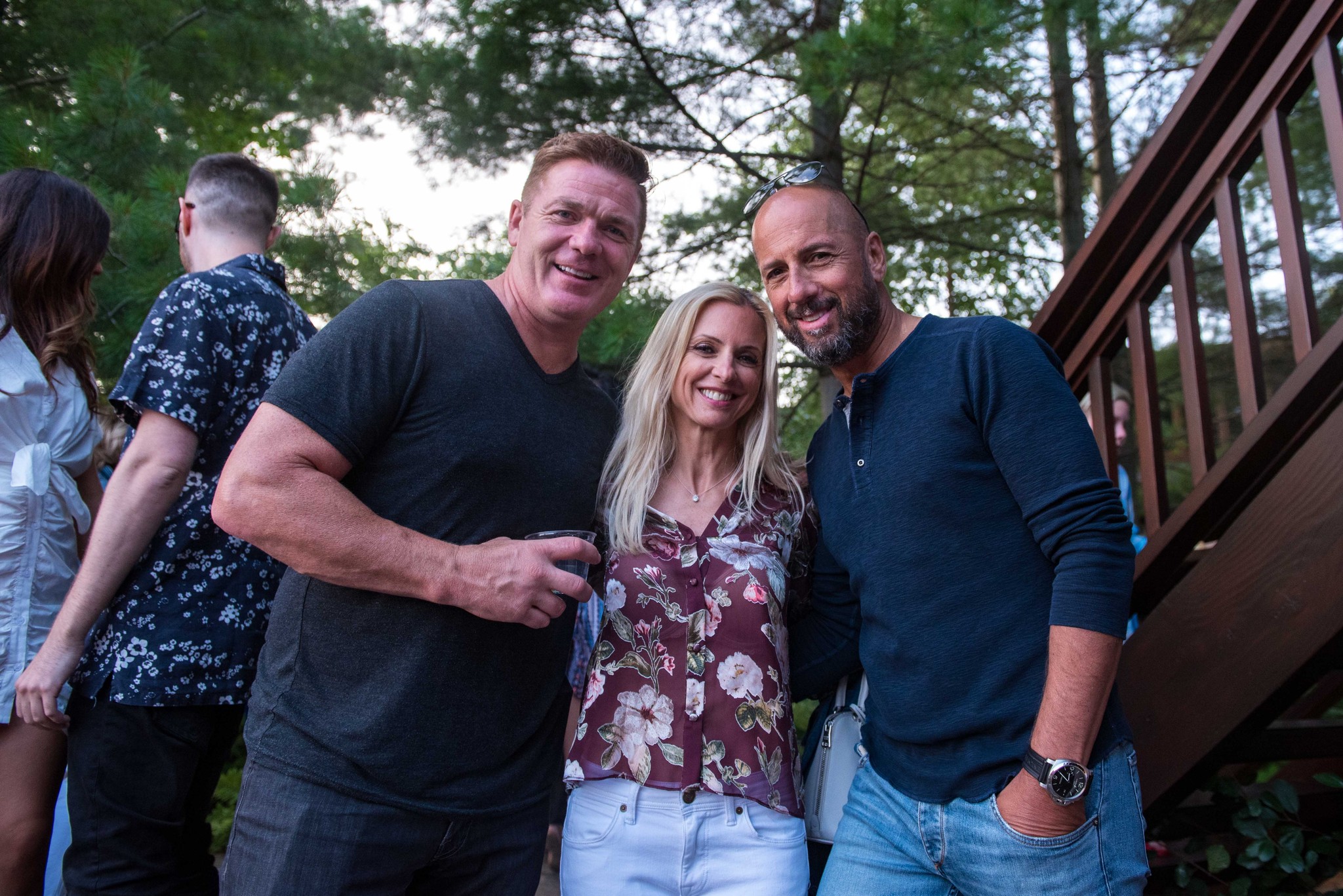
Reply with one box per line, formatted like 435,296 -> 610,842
0,166 -> 112,896
740,165 -> 1158,896
563,278 -> 819,896
1077,380 -> 1152,643
14,148 -> 319,895
214,127 -> 656,896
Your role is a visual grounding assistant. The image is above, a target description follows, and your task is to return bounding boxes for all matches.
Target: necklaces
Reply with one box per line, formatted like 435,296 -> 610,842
667,459 -> 740,501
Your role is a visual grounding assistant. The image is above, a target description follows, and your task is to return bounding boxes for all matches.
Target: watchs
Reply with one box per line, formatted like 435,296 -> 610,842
1021,749 -> 1095,808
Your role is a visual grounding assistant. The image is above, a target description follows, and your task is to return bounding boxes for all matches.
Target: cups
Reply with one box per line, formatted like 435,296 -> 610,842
525,530 -> 597,594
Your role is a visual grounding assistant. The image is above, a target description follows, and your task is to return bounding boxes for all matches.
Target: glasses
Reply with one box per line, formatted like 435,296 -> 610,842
743,161 -> 869,246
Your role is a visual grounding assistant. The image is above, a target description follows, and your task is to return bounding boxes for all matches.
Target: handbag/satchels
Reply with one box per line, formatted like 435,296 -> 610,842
802,669 -> 872,843
